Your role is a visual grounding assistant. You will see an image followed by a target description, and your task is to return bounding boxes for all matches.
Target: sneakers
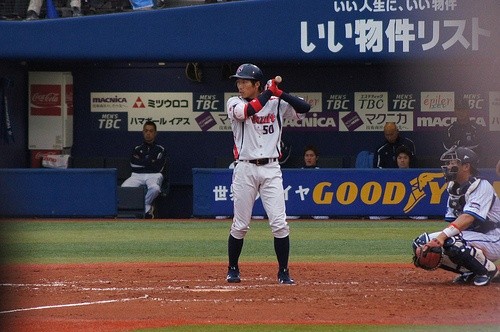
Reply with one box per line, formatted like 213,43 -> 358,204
277,268 -> 295,284
454,273 -> 475,284
473,265 -> 500,286
226,266 -> 241,283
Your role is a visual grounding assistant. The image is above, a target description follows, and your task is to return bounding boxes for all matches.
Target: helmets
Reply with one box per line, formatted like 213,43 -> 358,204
229,64 -> 264,81
440,147 -> 479,165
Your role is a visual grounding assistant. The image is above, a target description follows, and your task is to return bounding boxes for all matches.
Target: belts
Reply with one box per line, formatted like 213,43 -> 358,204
239,158 -> 276,165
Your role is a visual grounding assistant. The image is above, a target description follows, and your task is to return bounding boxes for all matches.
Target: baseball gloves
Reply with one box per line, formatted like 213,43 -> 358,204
412,237 -> 443,271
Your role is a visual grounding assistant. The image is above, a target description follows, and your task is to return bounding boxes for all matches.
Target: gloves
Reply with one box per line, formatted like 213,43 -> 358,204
267,78 -> 284,97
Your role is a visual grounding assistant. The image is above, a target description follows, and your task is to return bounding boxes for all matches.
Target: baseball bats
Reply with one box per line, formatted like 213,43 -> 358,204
273,75 -> 283,85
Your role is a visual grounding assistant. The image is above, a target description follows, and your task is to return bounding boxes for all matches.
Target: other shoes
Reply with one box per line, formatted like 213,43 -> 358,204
146,205 -> 156,219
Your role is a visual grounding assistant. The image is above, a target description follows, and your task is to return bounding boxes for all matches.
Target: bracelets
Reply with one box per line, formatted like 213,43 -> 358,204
443,224 -> 461,237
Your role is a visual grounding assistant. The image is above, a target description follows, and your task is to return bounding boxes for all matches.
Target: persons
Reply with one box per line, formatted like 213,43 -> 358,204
298,143 -> 322,169
22,0 -> 85,20
225,63 -> 311,285
440,98 -> 482,168
377,121 -> 418,169
411,143 -> 500,287
119,120 -> 170,220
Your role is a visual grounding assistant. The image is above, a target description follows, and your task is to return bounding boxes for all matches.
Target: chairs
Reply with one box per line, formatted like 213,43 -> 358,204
356,151 -> 373,169
